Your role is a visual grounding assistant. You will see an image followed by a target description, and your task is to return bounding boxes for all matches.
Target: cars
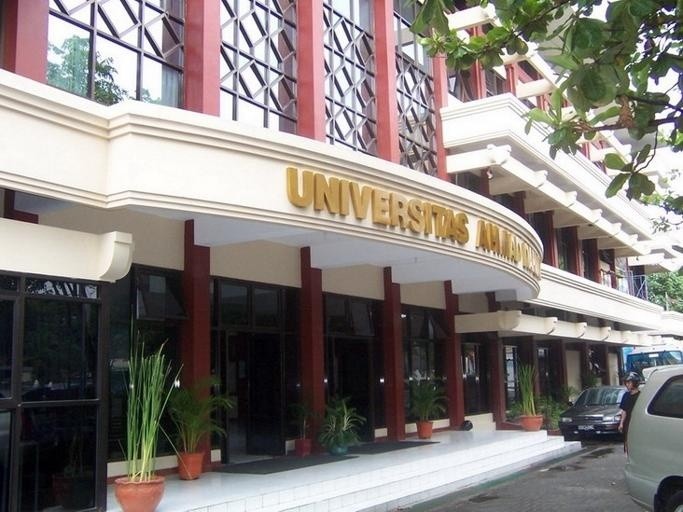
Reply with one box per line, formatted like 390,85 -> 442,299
559,385 -> 628,441
624,367 -> 683,512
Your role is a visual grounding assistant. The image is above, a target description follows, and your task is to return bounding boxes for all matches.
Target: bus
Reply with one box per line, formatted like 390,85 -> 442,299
622,347 -> 682,385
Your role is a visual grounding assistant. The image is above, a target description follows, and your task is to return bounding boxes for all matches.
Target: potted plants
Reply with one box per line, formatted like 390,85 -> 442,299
545,385 -> 579,435
114,302 -> 184,511
403,373 -> 450,438
316,393 -> 367,456
161,374 -> 237,480
285,399 -> 320,457
516,364 -> 543,431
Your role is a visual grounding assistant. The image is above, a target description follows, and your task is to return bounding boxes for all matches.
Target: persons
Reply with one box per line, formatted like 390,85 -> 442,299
618,372 -> 641,457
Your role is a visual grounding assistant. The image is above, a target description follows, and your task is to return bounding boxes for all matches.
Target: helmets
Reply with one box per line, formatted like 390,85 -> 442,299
621,371 -> 640,382
